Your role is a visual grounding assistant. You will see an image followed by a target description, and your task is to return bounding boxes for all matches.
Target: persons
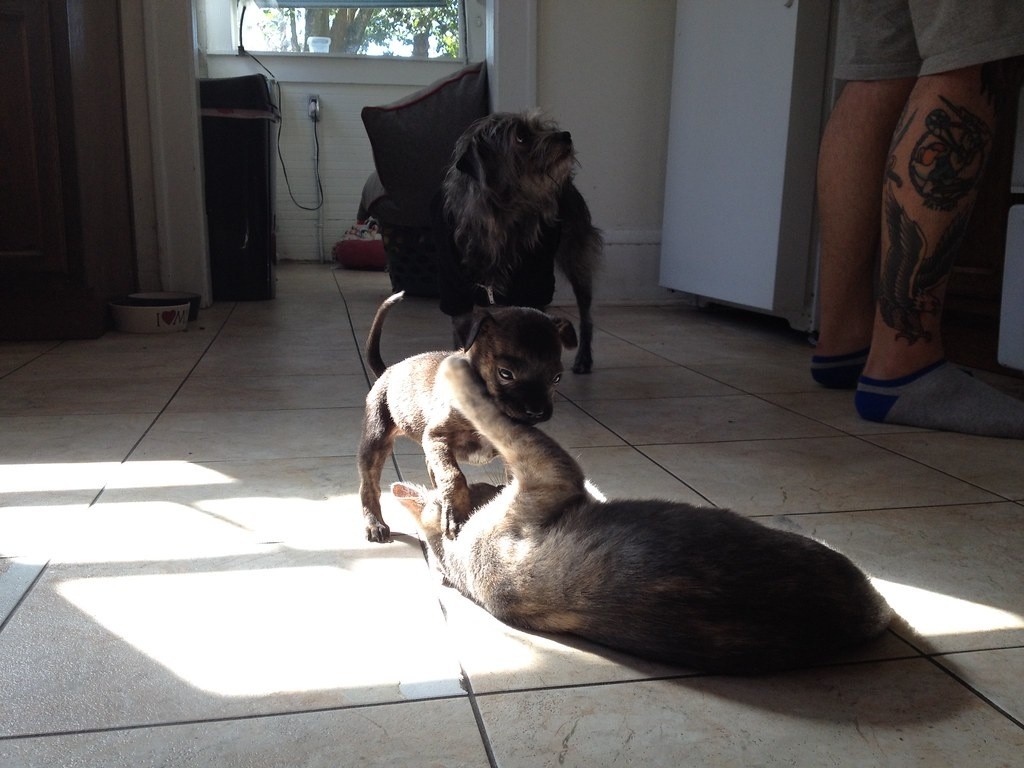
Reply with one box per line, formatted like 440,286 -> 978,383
807,1 -> 1024,436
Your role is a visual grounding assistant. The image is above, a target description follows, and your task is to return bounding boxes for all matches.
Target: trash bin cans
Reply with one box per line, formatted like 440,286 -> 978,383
198,71 -> 278,302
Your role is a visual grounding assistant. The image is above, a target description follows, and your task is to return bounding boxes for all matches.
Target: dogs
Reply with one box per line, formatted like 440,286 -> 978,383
429,107 -> 606,374
357,289 -> 579,543
390,354 -> 893,673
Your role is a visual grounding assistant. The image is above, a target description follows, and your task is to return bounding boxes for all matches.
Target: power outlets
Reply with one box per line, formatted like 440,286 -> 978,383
308,94 -> 320,119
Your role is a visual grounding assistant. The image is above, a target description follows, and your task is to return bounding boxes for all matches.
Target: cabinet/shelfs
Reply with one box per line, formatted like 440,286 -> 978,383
0,0 -> 136,343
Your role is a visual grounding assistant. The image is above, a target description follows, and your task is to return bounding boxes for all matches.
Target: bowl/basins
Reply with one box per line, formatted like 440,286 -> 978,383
108,300 -> 191,334
127,291 -> 202,321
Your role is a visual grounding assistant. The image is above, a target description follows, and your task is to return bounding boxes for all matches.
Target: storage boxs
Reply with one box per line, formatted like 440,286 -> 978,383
307,35 -> 331,52
